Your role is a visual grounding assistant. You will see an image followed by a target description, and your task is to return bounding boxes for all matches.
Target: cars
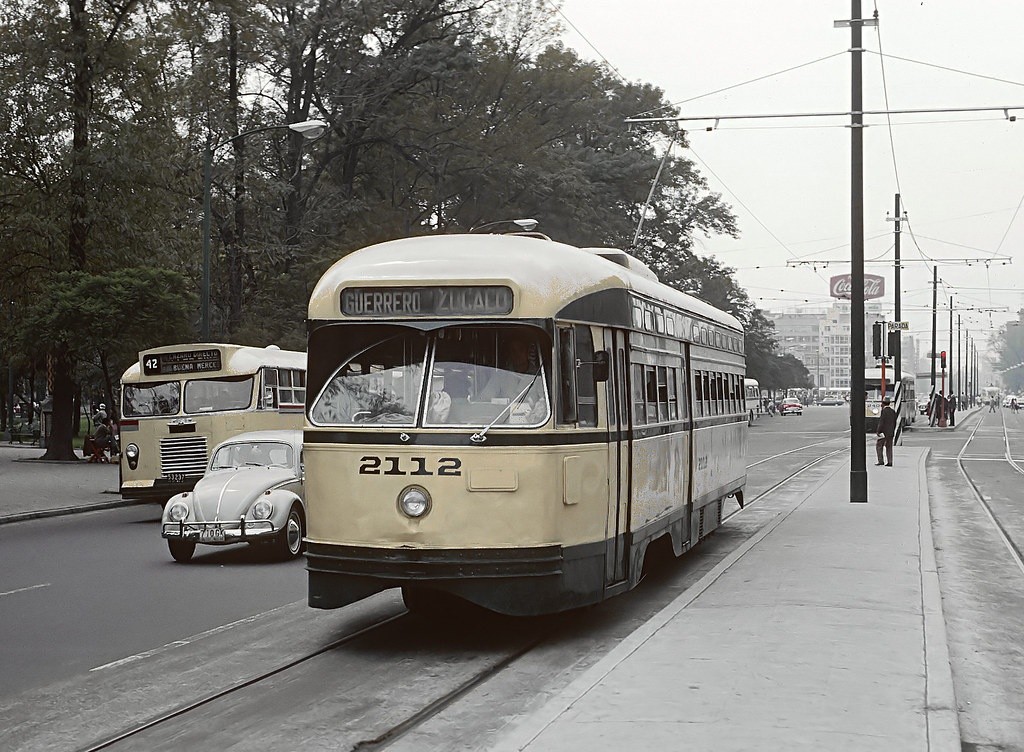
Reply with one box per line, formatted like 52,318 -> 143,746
918,396 -> 930,415
1003,395 -> 1024,409
915,393 -> 924,404
779,398 -> 804,416
816,397 -> 845,406
160,430 -> 305,564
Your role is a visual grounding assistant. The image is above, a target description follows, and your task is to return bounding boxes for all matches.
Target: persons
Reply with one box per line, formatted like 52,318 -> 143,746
767,401 -> 774,417
988,396 -> 996,413
476,336 -> 543,403
948,390 -> 956,426
936,390 -> 950,420
875,396 -> 897,466
1010,398 -> 1019,414
977,395 -> 981,407
92,403 -> 117,452
93,418 -> 120,453
961,392 -> 966,411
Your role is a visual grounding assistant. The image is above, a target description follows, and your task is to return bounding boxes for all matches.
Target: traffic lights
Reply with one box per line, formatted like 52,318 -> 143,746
941,351 -> 947,368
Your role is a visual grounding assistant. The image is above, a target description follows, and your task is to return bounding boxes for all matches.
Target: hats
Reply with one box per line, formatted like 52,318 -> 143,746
881,396 -> 892,402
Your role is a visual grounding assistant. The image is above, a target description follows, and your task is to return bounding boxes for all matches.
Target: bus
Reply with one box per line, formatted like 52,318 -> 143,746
118,341 -> 416,510
306,231 -> 750,615
865,364 -> 916,433
983,387 -> 1001,405
744,378 -> 761,426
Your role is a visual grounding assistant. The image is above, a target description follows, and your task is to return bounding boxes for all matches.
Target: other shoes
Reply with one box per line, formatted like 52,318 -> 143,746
875,462 -> 884,465
884,463 -> 892,466
